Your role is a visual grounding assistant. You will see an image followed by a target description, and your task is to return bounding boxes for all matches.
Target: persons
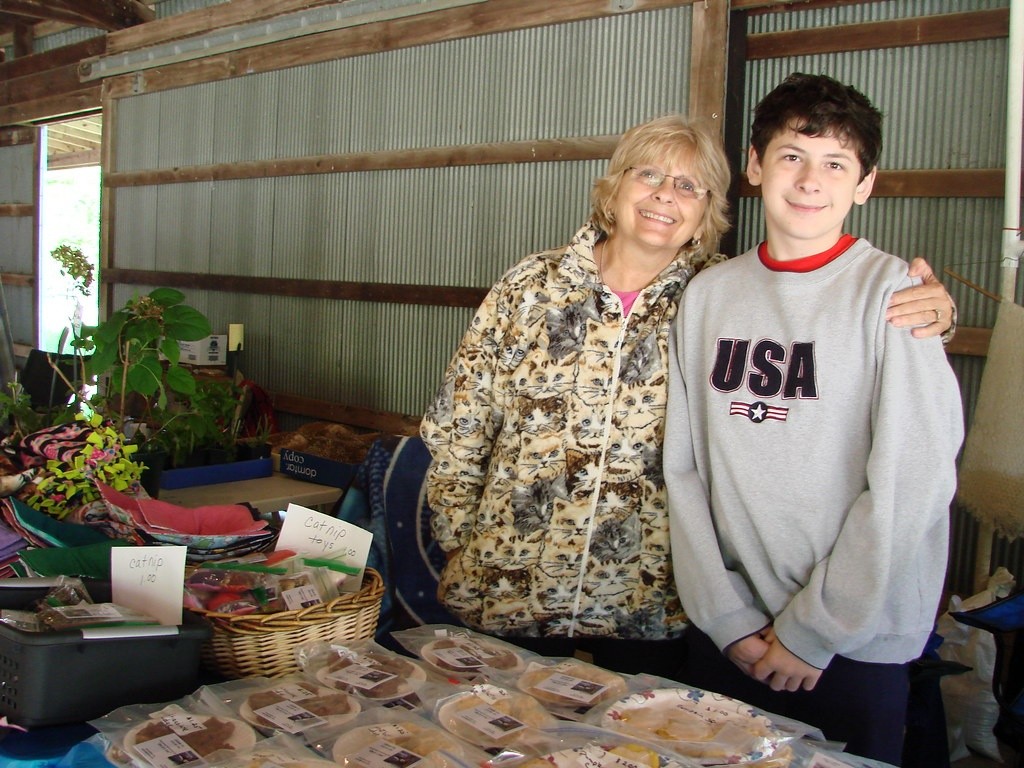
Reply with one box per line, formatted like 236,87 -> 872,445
417,112 -> 957,677
662,70 -> 965,767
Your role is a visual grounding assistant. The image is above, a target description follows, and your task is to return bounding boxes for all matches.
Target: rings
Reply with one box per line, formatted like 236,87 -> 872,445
934,309 -> 942,323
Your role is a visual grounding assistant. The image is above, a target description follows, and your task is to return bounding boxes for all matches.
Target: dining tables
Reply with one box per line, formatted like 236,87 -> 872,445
2,652 -> 905,768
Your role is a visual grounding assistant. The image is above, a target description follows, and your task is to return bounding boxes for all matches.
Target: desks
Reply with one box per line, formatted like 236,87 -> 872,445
158,475 -> 341,532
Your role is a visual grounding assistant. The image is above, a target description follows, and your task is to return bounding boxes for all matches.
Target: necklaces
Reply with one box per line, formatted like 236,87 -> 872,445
601,236 -> 609,287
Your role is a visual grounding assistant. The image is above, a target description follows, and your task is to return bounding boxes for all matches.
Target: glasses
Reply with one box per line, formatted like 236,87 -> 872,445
622,167 -> 710,199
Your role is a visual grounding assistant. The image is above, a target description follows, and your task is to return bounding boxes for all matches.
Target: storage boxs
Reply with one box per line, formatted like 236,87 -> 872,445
156,332 -> 227,366
182,552 -> 386,681
2,613 -> 210,727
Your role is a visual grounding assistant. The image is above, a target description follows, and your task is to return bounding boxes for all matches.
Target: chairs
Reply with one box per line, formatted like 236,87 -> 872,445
20,347 -> 94,422
